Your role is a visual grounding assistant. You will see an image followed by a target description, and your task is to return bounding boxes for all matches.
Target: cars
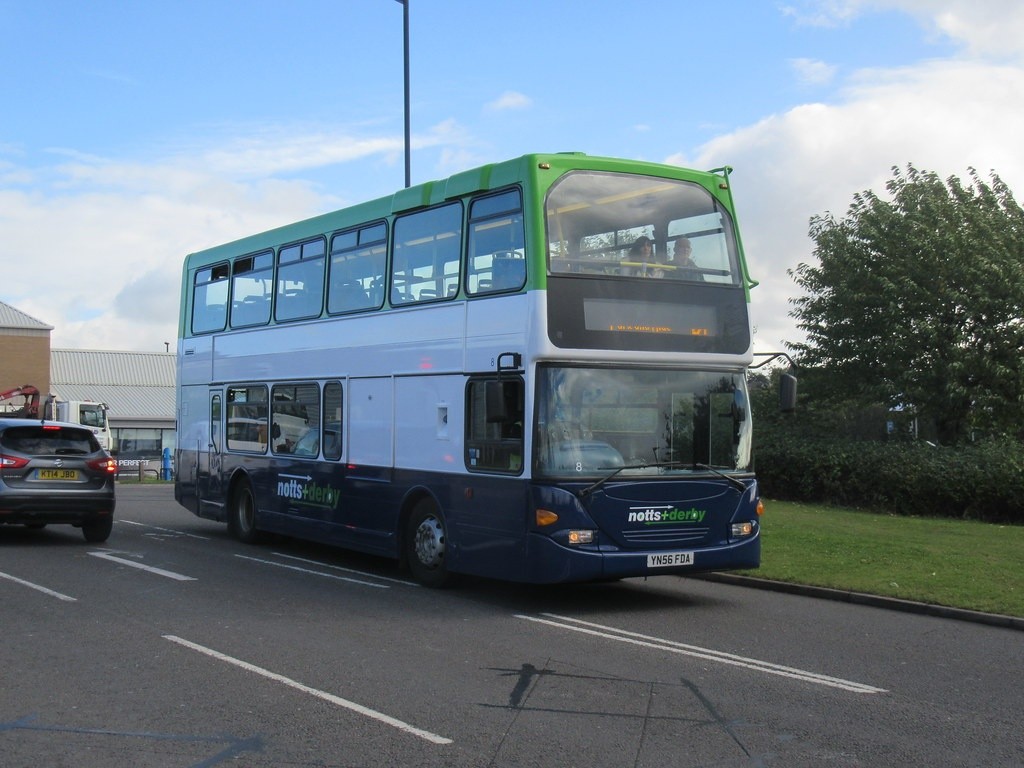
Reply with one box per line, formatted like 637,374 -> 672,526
476,437 -> 623,470
0,416 -> 119,543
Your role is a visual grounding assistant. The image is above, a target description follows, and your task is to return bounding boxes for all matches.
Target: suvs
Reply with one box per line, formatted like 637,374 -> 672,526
290,421 -> 343,462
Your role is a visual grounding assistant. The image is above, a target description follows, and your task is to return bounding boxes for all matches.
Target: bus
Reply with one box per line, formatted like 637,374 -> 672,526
175,149 -> 802,592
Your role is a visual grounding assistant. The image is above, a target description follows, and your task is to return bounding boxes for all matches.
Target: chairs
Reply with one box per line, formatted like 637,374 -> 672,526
206,247 -> 523,330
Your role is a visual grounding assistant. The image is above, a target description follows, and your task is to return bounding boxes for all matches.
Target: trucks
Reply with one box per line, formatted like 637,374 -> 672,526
181,416 -> 311,485
0,384 -> 113,460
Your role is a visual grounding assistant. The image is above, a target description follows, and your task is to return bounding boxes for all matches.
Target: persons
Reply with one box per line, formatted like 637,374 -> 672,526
662,238 -> 703,280
620,236 -> 664,278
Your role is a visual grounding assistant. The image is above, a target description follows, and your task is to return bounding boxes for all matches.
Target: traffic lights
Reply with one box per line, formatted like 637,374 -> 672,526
121,439 -> 132,452
